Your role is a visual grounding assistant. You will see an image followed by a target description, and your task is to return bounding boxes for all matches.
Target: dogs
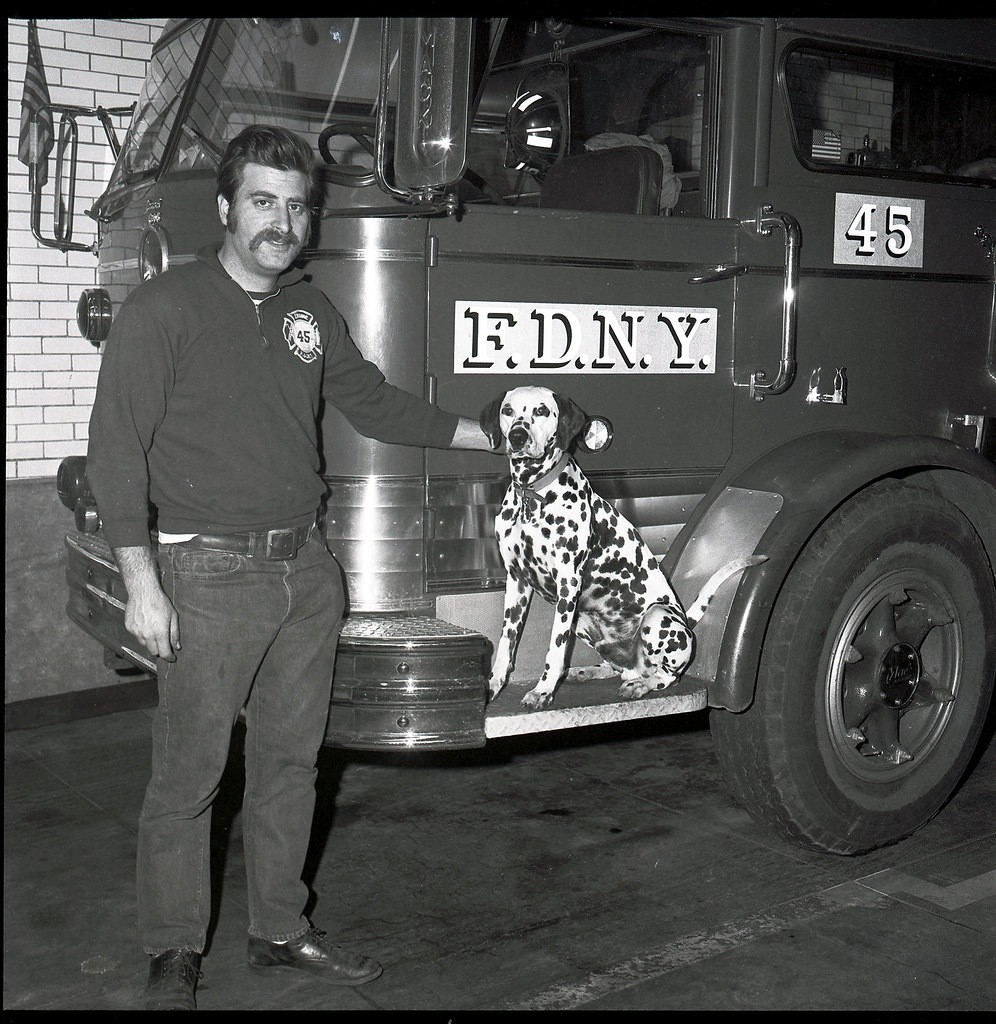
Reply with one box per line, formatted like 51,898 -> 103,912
478,386 -> 769,714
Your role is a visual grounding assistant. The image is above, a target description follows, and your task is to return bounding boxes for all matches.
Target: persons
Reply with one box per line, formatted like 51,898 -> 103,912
85,125 -> 506,1010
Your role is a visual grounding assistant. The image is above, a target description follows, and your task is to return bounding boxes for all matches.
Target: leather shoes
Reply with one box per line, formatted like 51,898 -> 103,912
142,946 -> 201,1010
247,926 -> 384,987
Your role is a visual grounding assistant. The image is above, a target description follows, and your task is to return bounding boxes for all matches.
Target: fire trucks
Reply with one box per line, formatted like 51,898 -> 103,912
29,15 -> 996,861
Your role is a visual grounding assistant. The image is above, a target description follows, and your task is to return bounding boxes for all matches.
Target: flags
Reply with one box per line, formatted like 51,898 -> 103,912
16,21 -> 56,189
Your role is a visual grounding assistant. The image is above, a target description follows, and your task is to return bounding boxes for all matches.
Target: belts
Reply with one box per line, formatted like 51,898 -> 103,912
161,522 -> 316,562
